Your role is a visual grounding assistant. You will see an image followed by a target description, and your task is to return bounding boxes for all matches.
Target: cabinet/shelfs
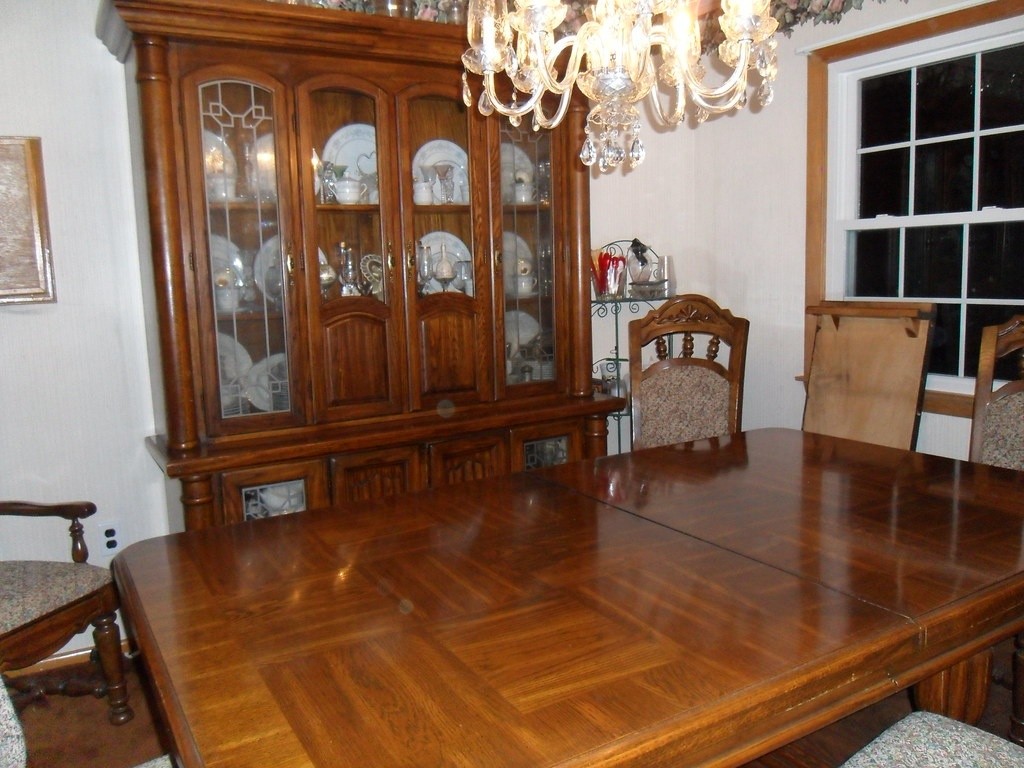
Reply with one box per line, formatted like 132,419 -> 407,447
92,0 -> 632,533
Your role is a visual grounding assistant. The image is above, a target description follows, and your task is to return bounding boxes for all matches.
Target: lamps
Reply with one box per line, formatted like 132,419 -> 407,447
460,0 -> 869,172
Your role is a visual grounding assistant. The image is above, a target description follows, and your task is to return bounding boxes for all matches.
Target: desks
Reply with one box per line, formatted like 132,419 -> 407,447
109,427 -> 1024,768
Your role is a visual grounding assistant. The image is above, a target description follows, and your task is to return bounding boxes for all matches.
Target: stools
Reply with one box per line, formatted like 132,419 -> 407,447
841,709 -> 1024,768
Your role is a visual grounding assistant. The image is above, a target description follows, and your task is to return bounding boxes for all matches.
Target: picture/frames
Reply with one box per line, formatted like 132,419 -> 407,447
2,133 -> 58,307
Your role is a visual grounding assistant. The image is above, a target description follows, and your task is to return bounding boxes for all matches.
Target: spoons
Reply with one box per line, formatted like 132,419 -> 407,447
590,249 -> 626,296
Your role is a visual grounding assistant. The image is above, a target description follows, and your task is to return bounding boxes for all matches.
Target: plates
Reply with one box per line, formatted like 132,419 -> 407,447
503,310 -> 553,382
322,123 -> 379,204
246,353 -> 290,411
203,129 -> 248,203
254,133 -> 275,191
252,233 -> 282,302
502,231 -> 539,297
217,332 -> 254,416
211,233 -> 245,303
412,138 -> 471,205
414,231 -> 471,289
499,142 -> 540,206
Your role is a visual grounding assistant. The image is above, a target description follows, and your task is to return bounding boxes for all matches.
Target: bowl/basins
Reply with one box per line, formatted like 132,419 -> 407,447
213,178 -> 236,199
217,290 -> 239,310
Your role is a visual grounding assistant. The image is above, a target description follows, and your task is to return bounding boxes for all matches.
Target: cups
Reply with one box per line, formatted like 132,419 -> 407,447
656,256 -> 678,296
515,184 -> 538,201
328,172 -> 366,203
516,276 -> 537,292
413,183 -> 434,206
599,363 -> 621,393
460,185 -> 469,202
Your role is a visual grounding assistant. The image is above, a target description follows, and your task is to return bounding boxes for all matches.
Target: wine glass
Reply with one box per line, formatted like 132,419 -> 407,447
419,246 -> 433,296
456,261 -> 471,293
420,165 -> 451,204
265,269 -> 282,313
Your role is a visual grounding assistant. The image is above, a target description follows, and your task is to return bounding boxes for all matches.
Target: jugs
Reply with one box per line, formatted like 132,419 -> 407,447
259,487 -> 302,510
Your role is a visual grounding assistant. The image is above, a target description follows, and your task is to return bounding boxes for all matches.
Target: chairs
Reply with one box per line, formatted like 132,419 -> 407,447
629,293 -> 751,454
0,500 -> 135,728
971,315 -> 1024,466
